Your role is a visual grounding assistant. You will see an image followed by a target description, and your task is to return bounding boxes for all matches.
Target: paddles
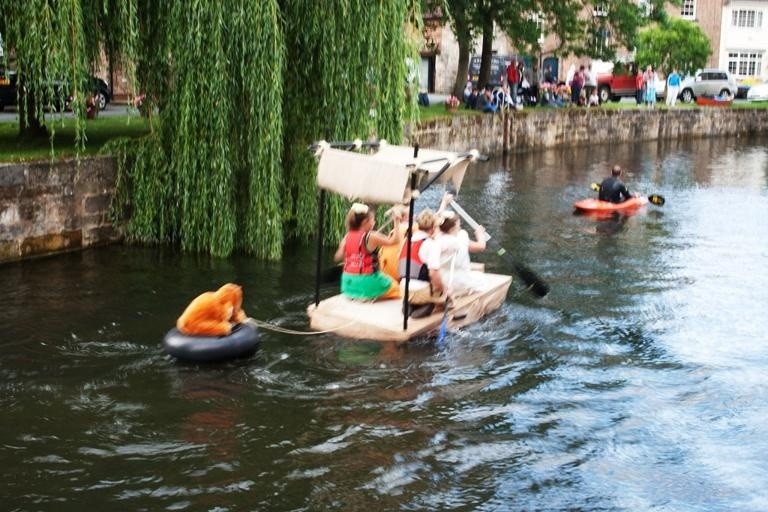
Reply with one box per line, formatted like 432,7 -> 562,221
590,182 -> 665,206
434,246 -> 459,346
451,200 -> 550,299
324,215 -> 394,281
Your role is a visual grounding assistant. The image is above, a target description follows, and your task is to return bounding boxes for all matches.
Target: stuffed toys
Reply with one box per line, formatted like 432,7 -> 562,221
176,284 -> 245,335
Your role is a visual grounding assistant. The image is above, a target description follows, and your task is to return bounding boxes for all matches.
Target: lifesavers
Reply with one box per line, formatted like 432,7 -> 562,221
163,316 -> 262,365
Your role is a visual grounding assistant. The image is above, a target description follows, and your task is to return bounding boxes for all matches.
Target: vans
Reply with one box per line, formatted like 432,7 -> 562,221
469,58 -> 537,92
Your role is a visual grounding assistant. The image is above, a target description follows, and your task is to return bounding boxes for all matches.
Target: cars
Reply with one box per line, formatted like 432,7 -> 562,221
129,92 -> 156,108
0,62 -> 110,110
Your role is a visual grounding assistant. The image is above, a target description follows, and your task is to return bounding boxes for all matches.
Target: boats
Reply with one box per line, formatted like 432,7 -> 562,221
573,191 -> 646,218
307,263 -> 513,343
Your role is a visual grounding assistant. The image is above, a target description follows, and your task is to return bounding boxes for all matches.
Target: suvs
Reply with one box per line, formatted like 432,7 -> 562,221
576,61 -> 648,104
654,69 -> 738,103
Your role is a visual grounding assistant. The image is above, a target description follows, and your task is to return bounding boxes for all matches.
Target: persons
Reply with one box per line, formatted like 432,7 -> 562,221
636,65 -> 656,109
666,68 -> 681,107
463,58 -> 602,113
598,165 -> 640,203
333,183 -> 487,317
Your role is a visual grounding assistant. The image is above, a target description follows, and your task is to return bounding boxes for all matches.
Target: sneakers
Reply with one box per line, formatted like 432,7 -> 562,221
402,299 -> 413,315
412,304 -> 434,318
